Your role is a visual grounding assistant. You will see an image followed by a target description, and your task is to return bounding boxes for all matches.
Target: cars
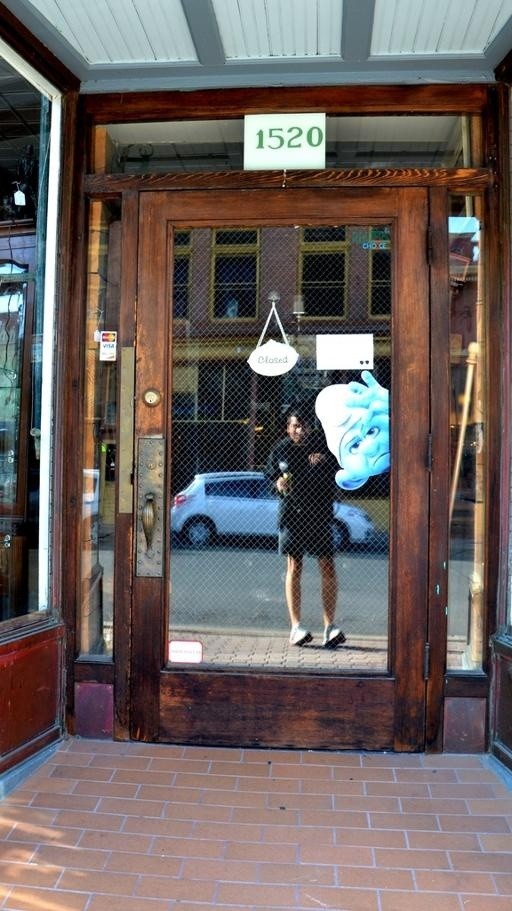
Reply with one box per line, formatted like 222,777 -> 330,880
167,470 -> 382,556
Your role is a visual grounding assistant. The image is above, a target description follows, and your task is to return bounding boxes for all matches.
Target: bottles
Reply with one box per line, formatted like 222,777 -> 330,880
282,466 -> 291,493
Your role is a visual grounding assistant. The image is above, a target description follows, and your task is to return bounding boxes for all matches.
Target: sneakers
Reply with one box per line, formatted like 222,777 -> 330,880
322,625 -> 346,649
289,627 -> 313,646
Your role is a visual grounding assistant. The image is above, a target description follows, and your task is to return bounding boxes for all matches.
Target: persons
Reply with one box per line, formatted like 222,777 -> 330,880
261,402 -> 351,651
